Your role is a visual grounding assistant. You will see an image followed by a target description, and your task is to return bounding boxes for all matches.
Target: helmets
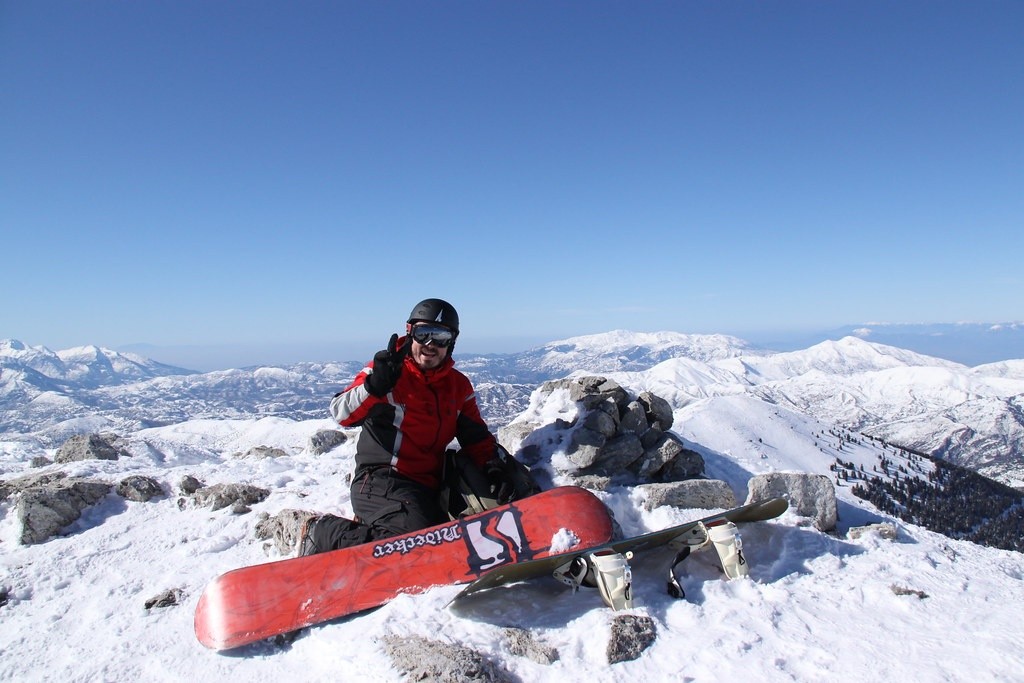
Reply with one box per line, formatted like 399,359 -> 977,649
406,298 -> 459,352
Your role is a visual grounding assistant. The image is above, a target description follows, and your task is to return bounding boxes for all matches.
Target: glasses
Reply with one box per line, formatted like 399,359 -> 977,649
410,324 -> 454,348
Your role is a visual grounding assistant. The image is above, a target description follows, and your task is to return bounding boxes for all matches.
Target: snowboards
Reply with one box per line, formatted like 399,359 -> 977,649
193,484 -> 613,650
453,497 -> 789,600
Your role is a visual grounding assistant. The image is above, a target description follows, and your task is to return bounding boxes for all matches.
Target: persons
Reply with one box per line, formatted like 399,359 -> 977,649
299,298 -> 611,589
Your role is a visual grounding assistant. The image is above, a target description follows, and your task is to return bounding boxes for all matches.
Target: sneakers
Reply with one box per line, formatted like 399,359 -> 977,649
298,513 -> 323,558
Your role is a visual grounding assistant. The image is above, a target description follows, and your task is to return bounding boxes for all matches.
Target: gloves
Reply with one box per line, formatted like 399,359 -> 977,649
485,464 -> 517,505
367,333 -> 413,396
